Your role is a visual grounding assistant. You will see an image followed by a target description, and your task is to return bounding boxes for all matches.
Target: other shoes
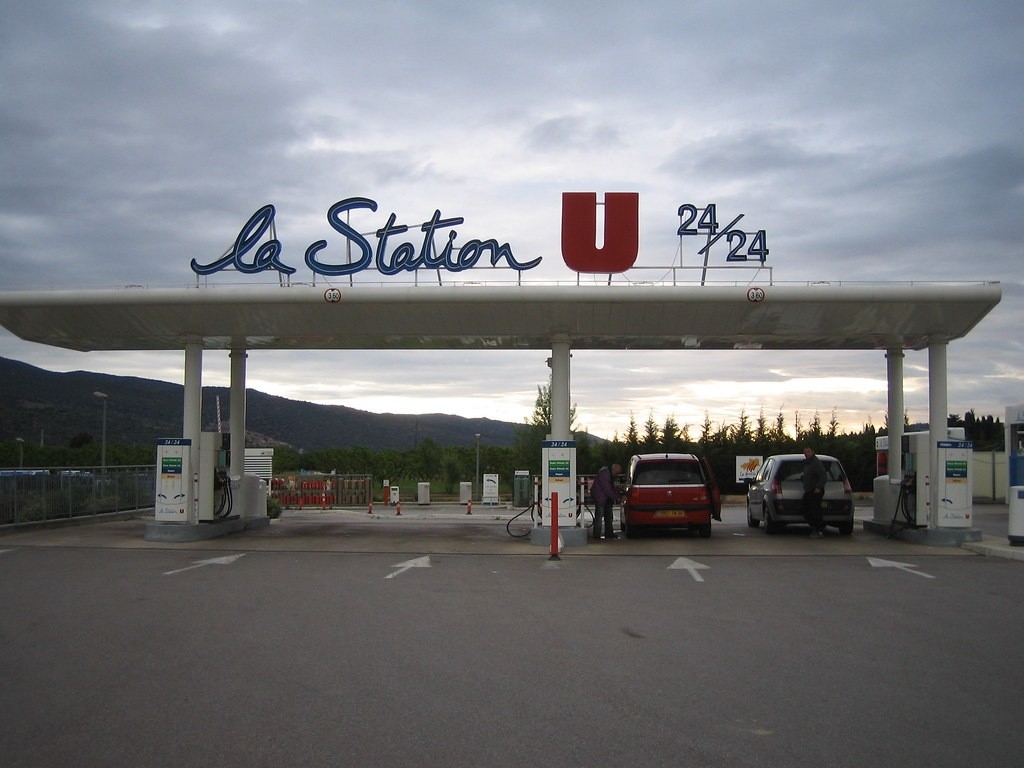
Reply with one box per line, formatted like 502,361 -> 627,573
809,529 -> 824,538
593,536 -> 604,541
605,534 -> 617,537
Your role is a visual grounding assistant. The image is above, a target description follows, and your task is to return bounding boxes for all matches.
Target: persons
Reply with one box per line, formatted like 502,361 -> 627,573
591,464 -> 622,541
801,444 -> 826,539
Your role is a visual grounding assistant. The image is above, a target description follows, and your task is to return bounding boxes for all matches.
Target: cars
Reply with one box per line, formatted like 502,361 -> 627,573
0,468 -> 149,481
744,454 -> 855,536
616,450 -> 723,539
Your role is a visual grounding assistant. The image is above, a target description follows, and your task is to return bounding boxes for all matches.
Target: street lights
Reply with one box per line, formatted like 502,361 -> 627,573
474,433 -> 481,501
93,390 -> 108,497
16,437 -> 24,470
